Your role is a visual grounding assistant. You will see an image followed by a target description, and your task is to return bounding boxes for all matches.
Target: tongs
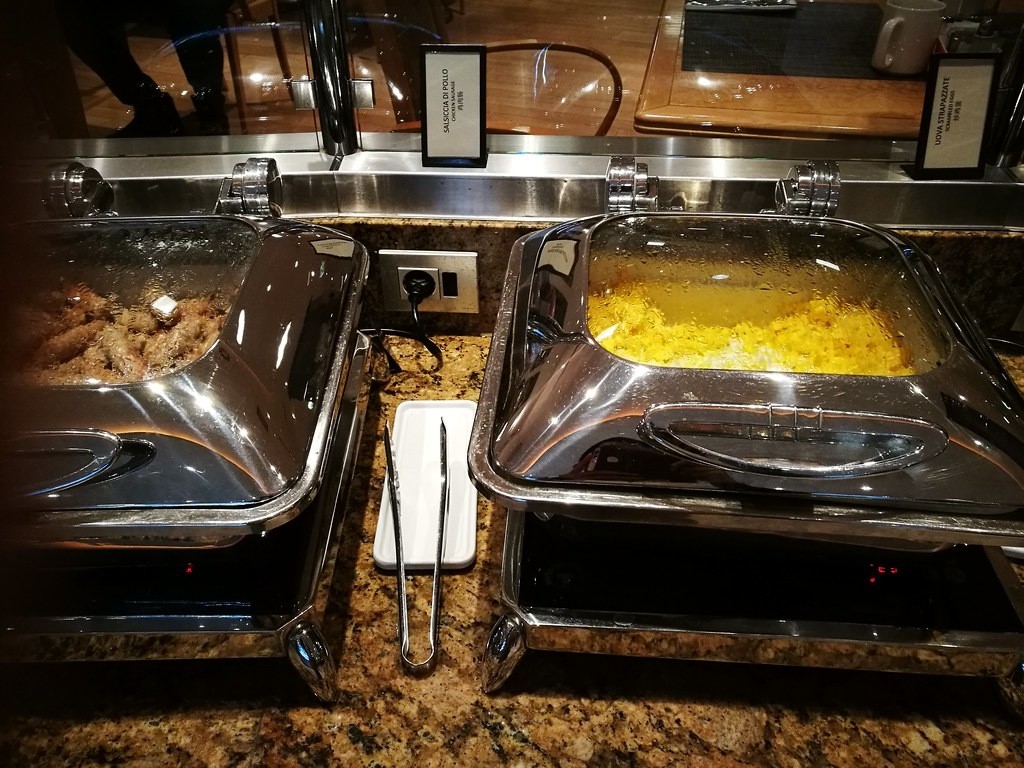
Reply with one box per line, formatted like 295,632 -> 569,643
384,417 -> 450,679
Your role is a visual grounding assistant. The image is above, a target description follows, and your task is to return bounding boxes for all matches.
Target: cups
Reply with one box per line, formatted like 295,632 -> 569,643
870,0 -> 948,77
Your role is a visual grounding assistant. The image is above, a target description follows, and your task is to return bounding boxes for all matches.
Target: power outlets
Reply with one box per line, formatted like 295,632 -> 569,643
380,248 -> 479,313
